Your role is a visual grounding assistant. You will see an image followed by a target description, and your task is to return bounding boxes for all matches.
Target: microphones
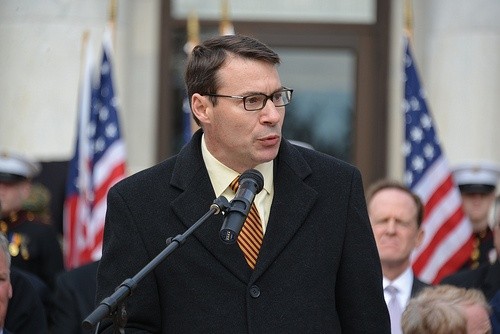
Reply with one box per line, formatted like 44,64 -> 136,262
218,168 -> 265,245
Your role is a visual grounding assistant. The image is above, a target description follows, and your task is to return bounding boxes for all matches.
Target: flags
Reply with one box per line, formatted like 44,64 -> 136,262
60,19 -> 126,275
402,36 -> 474,288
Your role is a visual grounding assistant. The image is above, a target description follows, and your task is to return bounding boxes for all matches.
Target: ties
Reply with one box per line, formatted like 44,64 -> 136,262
229,175 -> 263,270
384,285 -> 404,334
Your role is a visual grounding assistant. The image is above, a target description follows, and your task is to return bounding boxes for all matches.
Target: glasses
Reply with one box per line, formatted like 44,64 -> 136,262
198,83 -> 293,111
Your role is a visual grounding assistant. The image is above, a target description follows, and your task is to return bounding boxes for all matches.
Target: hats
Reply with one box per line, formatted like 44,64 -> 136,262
0,144 -> 41,187
451,164 -> 500,195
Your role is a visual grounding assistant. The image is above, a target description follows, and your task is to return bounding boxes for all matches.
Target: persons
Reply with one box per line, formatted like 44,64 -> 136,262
0,156 -> 61,274
450,163 -> 500,289
366,181 -> 433,334
400,284 -> 492,334
95,34 -> 391,334
